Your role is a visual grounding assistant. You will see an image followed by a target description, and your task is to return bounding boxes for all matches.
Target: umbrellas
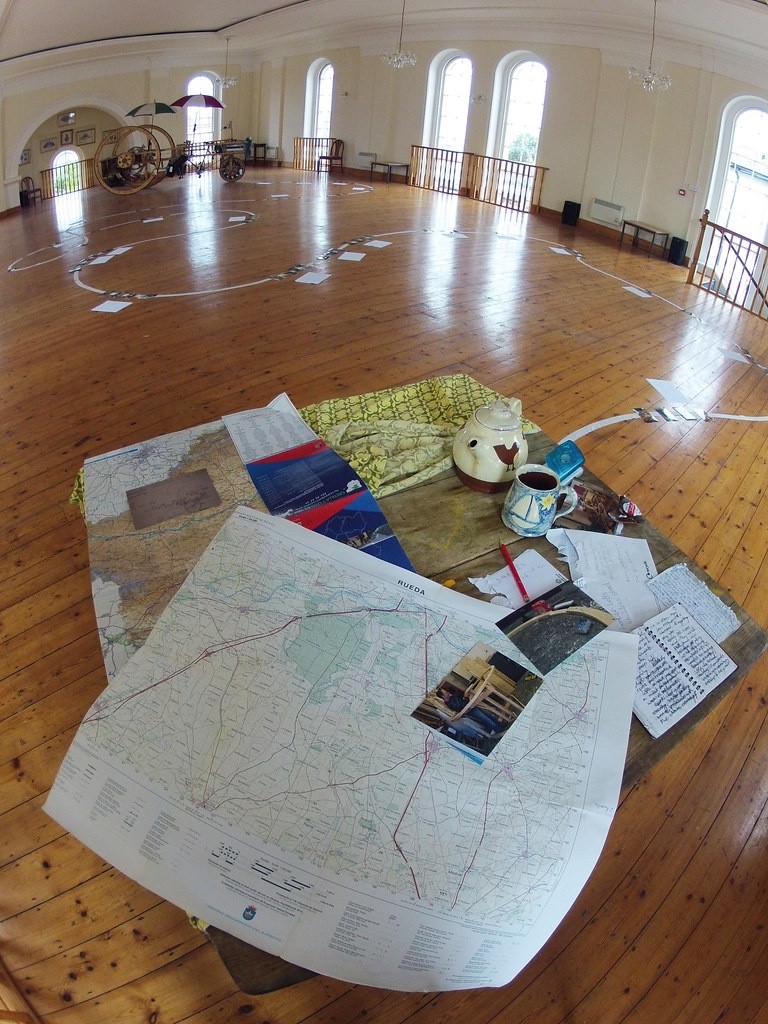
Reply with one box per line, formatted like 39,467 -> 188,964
170,94 -> 227,132
123,99 -> 176,133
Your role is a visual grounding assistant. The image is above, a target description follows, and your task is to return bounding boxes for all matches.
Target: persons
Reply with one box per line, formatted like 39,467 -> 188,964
437,688 -> 508,734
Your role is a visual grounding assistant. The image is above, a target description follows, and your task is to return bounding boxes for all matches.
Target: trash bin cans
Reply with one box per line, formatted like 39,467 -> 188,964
20,190 -> 30,206
668,236 -> 689,266
561,201 -> 581,227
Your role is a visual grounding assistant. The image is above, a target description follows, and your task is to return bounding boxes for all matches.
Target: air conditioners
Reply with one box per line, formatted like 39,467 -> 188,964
590,198 -> 624,226
266,147 -> 278,158
358,152 -> 377,168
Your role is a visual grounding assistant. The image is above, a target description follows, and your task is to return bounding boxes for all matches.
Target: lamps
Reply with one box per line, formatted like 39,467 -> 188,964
214,36 -> 239,90
625,0 -> 673,93
379,0 -> 418,69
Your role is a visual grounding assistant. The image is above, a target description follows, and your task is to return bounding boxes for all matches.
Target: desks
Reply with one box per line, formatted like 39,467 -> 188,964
245,143 -> 266,167
621,219 -> 669,260
370,161 -> 410,186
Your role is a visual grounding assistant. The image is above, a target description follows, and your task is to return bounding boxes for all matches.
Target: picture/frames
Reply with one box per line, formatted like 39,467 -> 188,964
104,129 -> 121,145
56,111 -> 76,127
18,149 -> 31,166
39,137 -> 58,154
60,129 -> 74,145
76,127 -> 96,147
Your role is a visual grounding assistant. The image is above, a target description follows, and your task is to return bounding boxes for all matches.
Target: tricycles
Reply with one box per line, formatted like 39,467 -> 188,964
92,92 -> 246,194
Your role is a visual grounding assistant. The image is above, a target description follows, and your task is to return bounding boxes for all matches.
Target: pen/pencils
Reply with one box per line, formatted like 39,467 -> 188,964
499,540 -> 531,604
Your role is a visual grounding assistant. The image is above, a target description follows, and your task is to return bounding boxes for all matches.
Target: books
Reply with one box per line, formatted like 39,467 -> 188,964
628,602 -> 739,739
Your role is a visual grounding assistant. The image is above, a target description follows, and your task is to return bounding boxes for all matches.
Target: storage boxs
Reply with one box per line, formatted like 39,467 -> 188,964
542,439 -> 585,486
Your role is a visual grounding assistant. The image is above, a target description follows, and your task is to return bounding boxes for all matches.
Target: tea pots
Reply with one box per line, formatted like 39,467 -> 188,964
452,398 -> 527,496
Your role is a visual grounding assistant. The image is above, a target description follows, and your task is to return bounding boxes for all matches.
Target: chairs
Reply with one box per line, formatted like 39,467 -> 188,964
20,176 -> 43,207
317,139 -> 344,176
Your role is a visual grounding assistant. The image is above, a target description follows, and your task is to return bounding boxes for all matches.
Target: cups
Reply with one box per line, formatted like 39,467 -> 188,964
502,462 -> 577,537
